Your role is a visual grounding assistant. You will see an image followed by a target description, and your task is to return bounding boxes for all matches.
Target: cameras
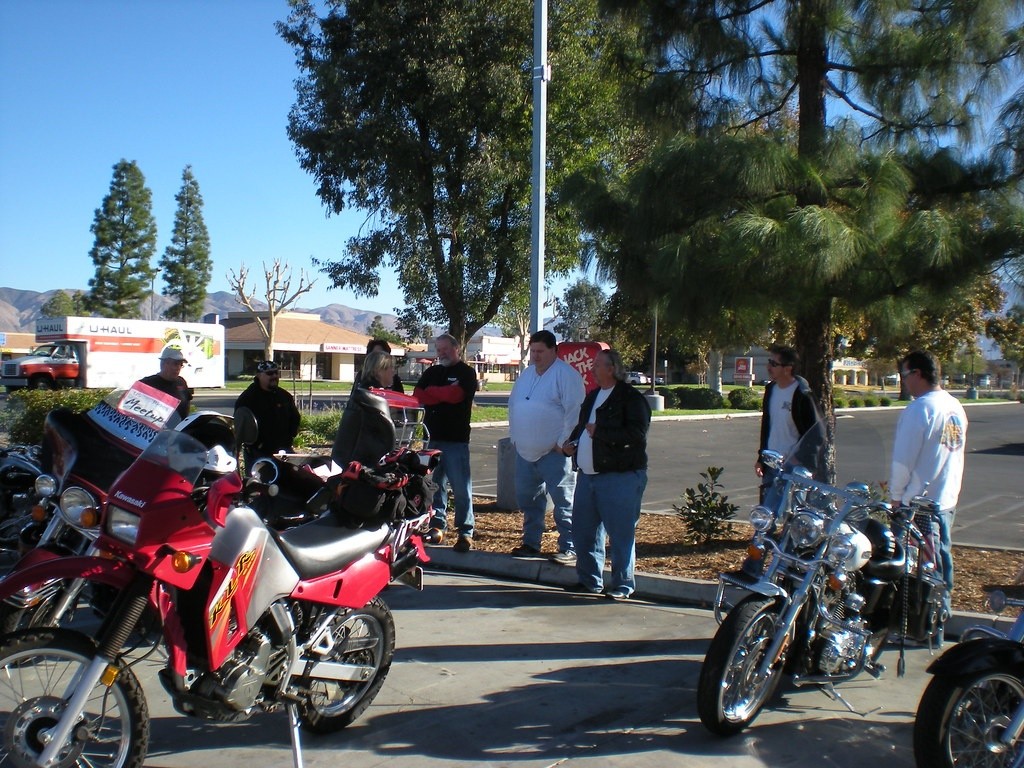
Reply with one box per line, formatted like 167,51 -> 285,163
568,439 -> 579,447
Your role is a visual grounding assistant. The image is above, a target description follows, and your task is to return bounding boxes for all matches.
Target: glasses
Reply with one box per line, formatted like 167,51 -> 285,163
898,369 -> 915,380
768,358 -> 793,368
259,370 -> 279,376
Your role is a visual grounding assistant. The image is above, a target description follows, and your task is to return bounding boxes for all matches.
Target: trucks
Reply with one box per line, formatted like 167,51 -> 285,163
0,315 -> 226,393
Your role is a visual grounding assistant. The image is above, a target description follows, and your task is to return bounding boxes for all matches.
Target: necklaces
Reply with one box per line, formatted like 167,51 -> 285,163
525,374 -> 541,400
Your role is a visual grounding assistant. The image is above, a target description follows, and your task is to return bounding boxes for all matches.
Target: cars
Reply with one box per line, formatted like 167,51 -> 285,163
624,371 -> 665,385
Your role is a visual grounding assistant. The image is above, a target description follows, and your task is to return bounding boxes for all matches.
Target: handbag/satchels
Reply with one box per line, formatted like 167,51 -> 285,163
245,446 -> 441,531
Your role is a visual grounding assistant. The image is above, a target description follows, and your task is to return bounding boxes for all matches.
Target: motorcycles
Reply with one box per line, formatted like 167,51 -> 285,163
696,414 -> 955,740
913,567 -> 1024,768
1,377 -> 446,768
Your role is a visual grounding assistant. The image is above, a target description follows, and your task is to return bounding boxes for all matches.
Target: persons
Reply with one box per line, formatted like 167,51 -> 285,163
413,334 -> 475,548
138,347 -> 190,421
754,342 -> 826,509
890,349 -> 968,648
233,360 -> 301,478
509,330 -> 586,563
562,349 -> 652,599
351,338 -> 405,395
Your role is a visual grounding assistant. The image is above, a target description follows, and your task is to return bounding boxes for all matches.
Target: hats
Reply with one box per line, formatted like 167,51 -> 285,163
257,361 -> 281,372
159,346 -> 188,363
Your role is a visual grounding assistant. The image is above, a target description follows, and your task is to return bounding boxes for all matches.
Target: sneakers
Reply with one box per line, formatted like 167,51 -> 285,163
548,549 -> 577,564
512,544 -> 540,557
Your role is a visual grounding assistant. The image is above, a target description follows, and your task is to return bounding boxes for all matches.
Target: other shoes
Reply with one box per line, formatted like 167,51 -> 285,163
422,527 -> 446,544
725,568 -> 759,585
453,536 -> 473,551
605,590 -> 626,600
892,628 -> 941,649
564,581 -> 592,593
